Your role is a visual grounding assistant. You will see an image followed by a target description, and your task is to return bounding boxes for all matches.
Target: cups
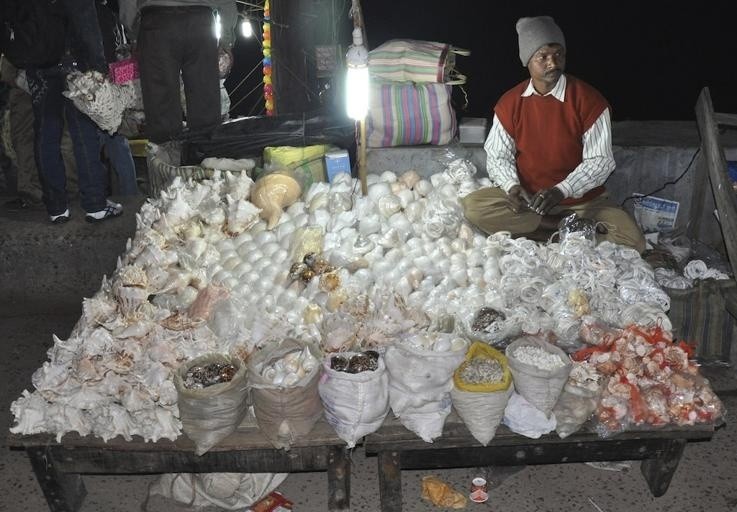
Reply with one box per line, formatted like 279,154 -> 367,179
470,478 -> 489,503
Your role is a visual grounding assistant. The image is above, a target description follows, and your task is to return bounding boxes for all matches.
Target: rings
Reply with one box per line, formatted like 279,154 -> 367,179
539,194 -> 544,199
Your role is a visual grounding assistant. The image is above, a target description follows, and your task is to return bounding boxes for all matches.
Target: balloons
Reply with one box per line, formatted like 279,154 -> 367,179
262,0 -> 275,118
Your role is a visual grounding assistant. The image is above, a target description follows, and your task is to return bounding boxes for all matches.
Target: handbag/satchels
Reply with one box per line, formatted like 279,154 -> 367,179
354,81 -> 457,148
61,70 -> 137,136
108,57 -> 140,84
0,1 -> 32,70
365,40 -> 471,86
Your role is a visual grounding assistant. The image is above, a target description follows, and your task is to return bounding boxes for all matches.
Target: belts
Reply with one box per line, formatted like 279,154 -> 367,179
140,5 -> 212,17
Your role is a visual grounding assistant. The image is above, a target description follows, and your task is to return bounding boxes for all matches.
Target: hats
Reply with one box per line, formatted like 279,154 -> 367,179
515,16 -> 567,68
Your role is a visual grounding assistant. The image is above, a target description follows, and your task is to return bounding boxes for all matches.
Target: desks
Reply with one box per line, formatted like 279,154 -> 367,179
8,426 -> 357,511
367,409 -> 723,511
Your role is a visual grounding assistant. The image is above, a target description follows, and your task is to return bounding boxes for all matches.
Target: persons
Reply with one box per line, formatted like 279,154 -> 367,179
0,1 -> 124,224
94,1 -> 140,196
117,0 -> 238,145
460,15 -> 646,255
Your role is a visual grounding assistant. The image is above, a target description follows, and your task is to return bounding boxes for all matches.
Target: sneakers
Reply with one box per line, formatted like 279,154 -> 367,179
49,209 -> 70,223
84,200 -> 123,221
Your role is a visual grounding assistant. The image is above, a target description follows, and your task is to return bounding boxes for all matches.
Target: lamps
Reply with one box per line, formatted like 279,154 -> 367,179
240,15 -> 252,38
344,27 -> 373,121
